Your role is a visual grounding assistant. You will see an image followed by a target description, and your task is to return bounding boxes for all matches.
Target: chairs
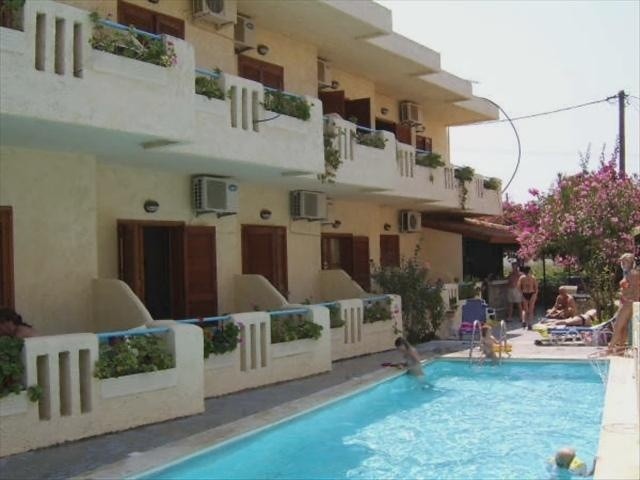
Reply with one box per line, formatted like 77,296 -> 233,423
458,295 -> 621,346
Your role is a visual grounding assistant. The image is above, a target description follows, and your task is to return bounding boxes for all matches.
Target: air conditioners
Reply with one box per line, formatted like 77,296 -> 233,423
190,0 -> 236,30
319,57 -> 332,89
191,174 -> 239,219
232,11 -> 259,53
289,188 -> 329,221
401,100 -> 423,127
399,208 -> 423,235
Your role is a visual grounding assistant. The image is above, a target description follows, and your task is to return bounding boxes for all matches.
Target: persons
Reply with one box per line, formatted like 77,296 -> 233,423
394,337 -> 423,376
555,309 -> 598,325
551,448 -> 596,480
479,325 -> 502,359
517,266 -> 538,331
505,262 -> 525,323
606,253 -> 640,356
546,287 -> 575,320
0,309 -> 37,337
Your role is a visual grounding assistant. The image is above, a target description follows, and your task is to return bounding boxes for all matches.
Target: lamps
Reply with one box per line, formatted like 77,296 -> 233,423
258,43 -> 270,56
381,106 -> 390,116
383,222 -> 392,231
261,207 -> 272,221
332,218 -> 343,229
332,79 -> 341,89
143,198 -> 161,213
